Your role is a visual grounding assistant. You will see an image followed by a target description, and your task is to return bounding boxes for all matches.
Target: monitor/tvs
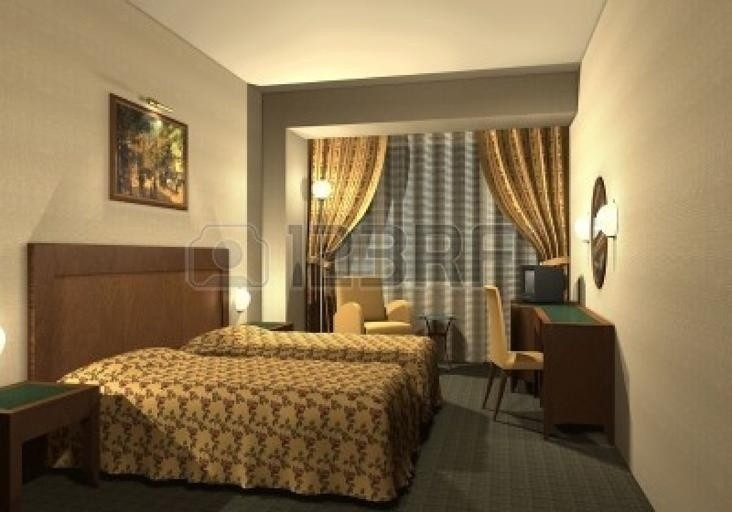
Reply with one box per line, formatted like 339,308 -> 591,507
517,265 -> 569,304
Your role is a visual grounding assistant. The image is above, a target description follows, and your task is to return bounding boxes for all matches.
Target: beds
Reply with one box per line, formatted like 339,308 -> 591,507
54,343 -> 426,504
180,324 -> 446,433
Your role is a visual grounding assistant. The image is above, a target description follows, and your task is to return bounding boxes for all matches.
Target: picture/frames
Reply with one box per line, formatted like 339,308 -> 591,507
104,92 -> 191,213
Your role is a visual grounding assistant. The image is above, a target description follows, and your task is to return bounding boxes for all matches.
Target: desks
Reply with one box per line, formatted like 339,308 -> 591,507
505,294 -> 619,450
414,313 -> 464,374
240,319 -> 293,331
1,379 -> 104,511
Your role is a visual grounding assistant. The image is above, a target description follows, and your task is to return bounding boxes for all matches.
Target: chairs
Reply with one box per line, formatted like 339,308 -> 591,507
329,273 -> 414,338
481,278 -> 548,425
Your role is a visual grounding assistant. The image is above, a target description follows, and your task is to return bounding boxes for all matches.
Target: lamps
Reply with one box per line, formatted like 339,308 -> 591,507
572,212 -> 591,244
233,285 -> 253,315
595,199 -> 618,242
308,176 -> 333,330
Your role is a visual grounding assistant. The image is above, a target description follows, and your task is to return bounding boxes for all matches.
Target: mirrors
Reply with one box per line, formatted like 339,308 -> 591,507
586,174 -> 611,290
